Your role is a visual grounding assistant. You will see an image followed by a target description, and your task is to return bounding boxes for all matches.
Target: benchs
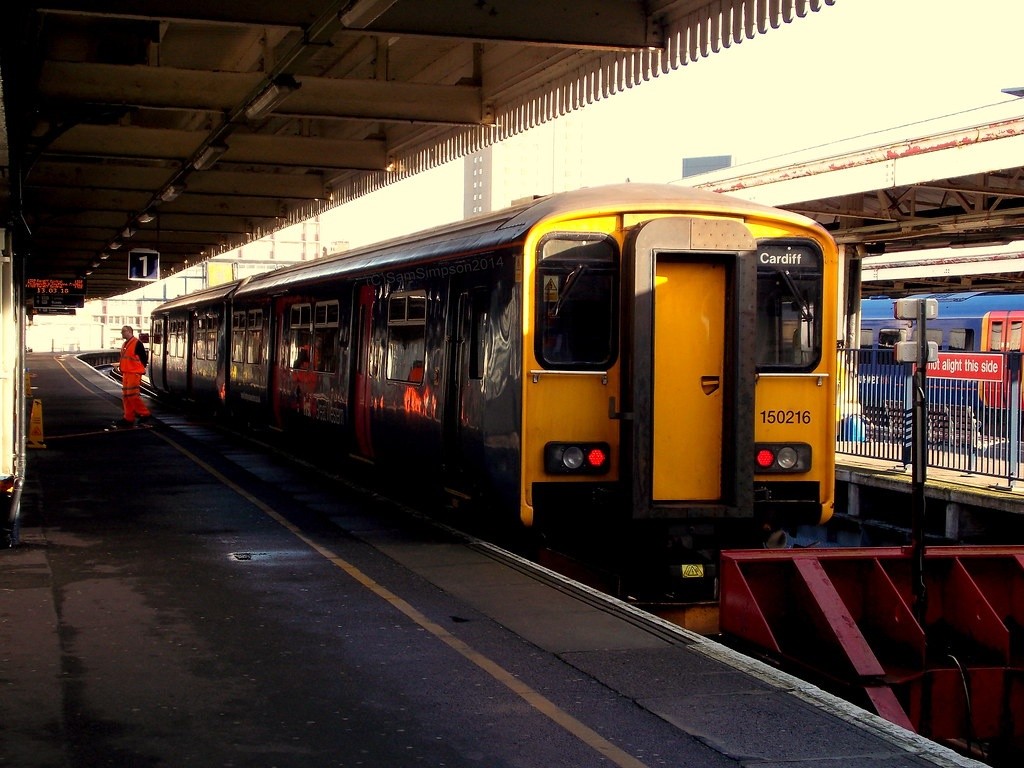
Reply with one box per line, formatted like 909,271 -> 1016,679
882,400 -> 981,452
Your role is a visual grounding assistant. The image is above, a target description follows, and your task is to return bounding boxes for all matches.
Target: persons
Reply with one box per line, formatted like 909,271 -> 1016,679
118,325 -> 152,423
292,334 -> 321,416
215,321 -> 229,399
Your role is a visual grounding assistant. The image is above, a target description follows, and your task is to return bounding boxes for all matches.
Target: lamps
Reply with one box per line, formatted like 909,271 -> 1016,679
85,210 -> 157,276
161,181 -> 187,202
193,144 -> 229,170
949,238 -> 1013,250
244,74 -> 303,120
339,0 -> 398,29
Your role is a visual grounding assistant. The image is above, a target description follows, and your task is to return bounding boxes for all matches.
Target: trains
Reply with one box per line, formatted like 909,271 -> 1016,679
146,178 -> 871,543
773,283 -> 1024,444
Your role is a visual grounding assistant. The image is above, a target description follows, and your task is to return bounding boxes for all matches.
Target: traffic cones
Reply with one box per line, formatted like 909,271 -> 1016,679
23,369 -> 33,399
26,399 -> 47,449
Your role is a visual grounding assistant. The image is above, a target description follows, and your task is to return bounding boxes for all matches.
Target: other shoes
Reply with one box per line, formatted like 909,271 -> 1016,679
113,419 -> 134,429
136,415 -> 152,424
